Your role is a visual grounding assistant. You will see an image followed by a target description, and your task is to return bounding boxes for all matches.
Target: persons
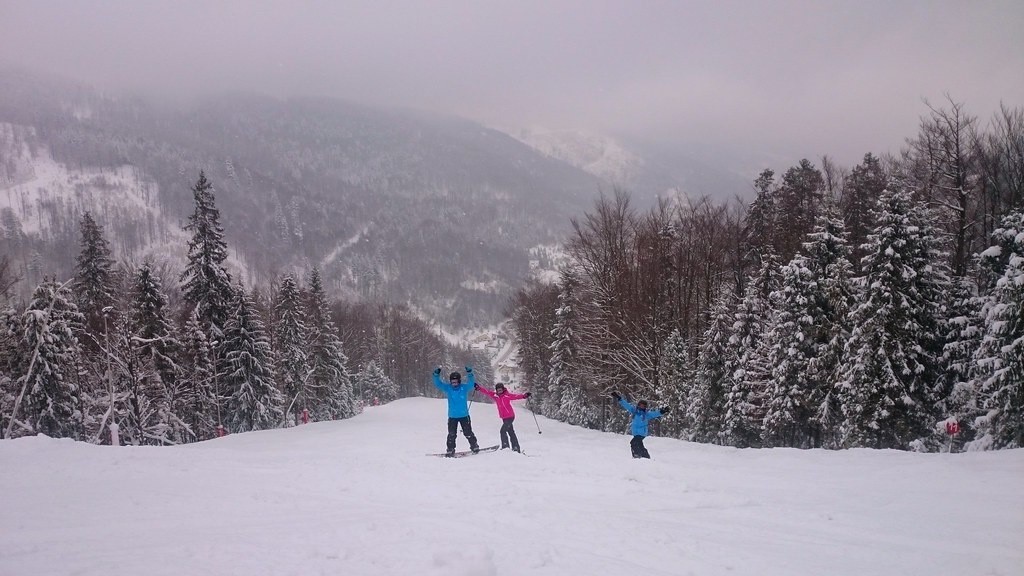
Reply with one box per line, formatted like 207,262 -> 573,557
473,382 -> 531,453
611,391 -> 670,459
432,365 -> 479,457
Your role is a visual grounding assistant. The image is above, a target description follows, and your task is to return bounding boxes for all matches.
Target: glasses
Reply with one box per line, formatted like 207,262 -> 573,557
451,377 -> 458,380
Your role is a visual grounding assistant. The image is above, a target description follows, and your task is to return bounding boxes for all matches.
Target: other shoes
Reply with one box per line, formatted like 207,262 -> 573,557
470,446 -> 480,454
446,448 -> 455,457
513,449 -> 520,453
500,447 -> 510,450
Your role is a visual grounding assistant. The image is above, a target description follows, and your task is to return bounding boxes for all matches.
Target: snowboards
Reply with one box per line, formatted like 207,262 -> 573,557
425,444 -> 500,458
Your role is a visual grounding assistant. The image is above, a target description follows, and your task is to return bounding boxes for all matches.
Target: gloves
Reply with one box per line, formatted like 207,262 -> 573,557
660,406 -> 670,414
433,368 -> 441,375
474,383 -> 479,390
525,393 -> 531,398
465,366 -> 472,372
611,392 -> 621,400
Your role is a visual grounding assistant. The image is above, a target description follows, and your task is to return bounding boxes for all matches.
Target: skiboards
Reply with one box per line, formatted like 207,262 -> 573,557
500,446 -> 532,457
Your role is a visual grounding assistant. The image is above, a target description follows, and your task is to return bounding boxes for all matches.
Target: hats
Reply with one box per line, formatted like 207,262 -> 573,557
639,400 -> 647,405
450,372 -> 461,381
495,383 -> 503,389
